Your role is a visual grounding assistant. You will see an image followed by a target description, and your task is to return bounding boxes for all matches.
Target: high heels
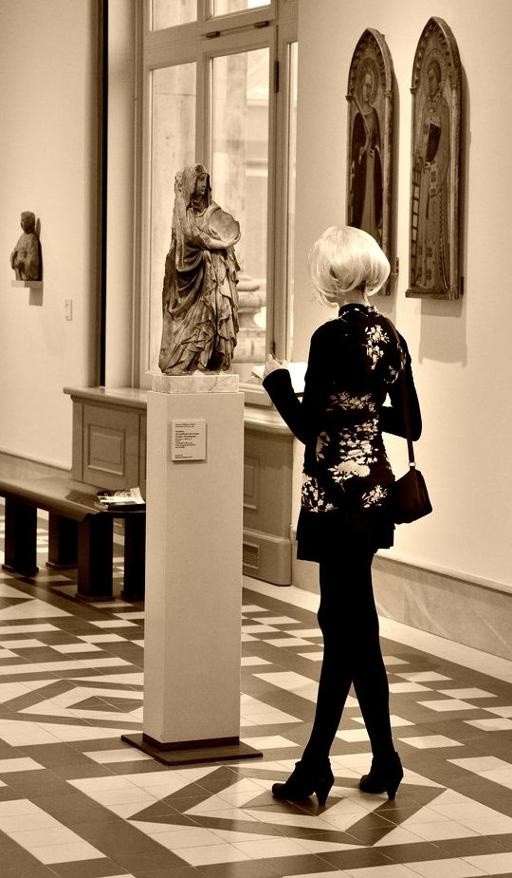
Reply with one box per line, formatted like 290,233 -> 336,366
272,757 -> 334,806
359,751 -> 403,801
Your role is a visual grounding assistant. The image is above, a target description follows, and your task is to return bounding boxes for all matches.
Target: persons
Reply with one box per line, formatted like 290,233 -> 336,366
349,58 -> 383,245
266,225 -> 424,809
411,58 -> 451,290
160,162 -> 243,375
8,209 -> 43,282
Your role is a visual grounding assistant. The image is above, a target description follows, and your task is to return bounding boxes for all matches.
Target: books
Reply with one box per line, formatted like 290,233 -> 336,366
93,484 -> 144,511
249,359 -> 310,393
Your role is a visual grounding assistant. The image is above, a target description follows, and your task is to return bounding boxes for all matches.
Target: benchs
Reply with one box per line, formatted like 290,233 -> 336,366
0,476 -> 145,602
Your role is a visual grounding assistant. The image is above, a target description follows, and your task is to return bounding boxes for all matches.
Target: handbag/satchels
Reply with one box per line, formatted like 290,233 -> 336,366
395,468 -> 432,525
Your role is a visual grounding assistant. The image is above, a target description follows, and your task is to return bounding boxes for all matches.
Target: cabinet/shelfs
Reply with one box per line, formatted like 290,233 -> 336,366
62,386 -> 293,586
